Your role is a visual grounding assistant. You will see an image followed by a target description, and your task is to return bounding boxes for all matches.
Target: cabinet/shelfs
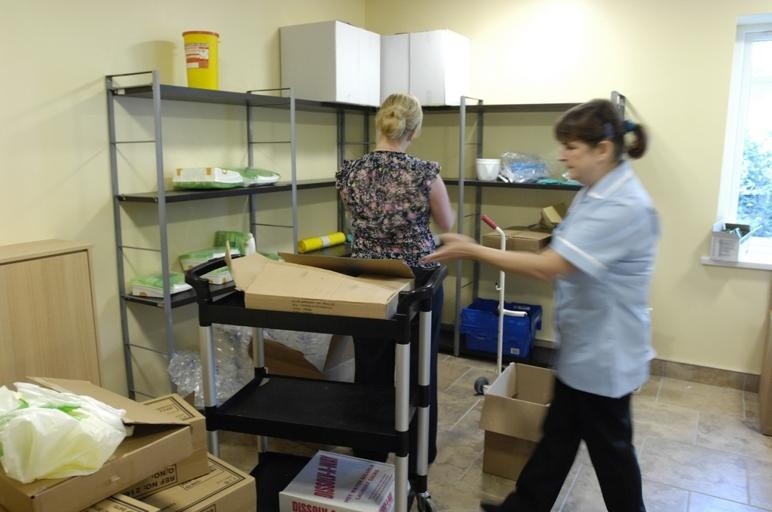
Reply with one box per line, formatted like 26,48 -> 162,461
0,72 -> 625,456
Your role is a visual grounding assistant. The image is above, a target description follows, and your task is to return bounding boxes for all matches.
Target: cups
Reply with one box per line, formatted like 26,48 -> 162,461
182,30 -> 220,91
476,158 -> 499,181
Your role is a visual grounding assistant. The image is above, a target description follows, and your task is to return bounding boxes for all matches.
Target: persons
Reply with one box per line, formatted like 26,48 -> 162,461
335,92 -> 456,465
419,96 -> 661,512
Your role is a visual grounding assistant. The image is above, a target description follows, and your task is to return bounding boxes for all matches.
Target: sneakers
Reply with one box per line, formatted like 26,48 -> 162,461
481,492 -> 516,512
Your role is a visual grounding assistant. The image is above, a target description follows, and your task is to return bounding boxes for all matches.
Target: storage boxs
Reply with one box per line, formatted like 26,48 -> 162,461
280,22 -> 381,109
0,379 -> 412,512
381,30 -> 465,109
480,362 -> 559,480
711,216 -> 762,264
